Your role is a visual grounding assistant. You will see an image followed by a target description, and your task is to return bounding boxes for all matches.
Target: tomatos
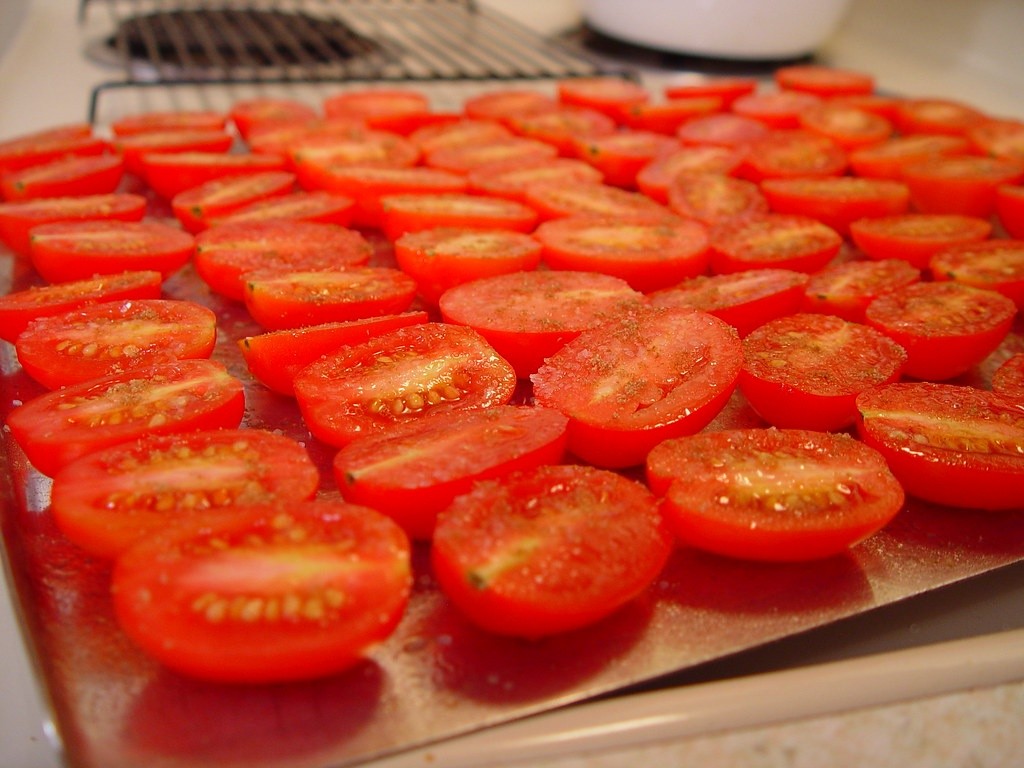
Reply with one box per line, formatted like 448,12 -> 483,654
0,65 -> 1024,686
